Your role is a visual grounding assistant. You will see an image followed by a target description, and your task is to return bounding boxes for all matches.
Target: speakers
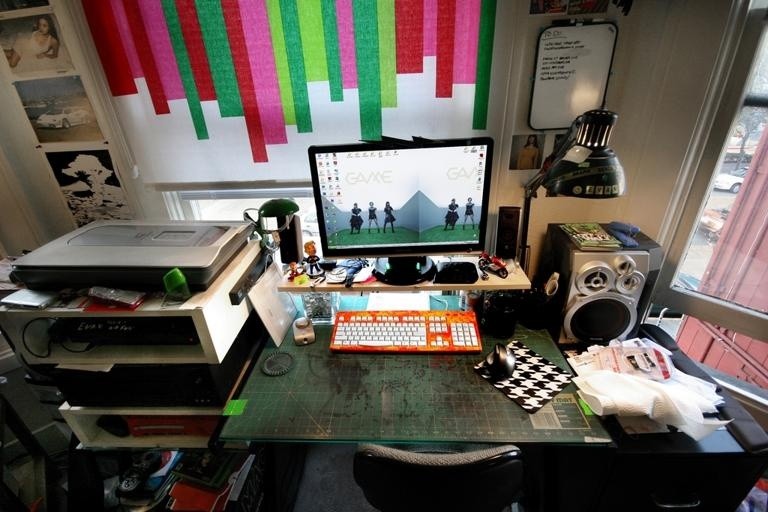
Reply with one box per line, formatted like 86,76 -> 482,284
494,206 -> 522,259
277,215 -> 303,263
533,223 -> 665,351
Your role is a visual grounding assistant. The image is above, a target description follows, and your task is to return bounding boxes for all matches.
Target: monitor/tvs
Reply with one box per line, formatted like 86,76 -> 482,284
308,136 -> 494,285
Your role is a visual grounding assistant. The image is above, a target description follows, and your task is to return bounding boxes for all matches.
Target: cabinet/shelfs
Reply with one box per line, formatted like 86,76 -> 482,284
521,323 -> 768,512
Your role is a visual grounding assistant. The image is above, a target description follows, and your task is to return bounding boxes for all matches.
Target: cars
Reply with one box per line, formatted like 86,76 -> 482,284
298,203 -> 319,239
698,200 -> 739,237
713,166 -> 752,195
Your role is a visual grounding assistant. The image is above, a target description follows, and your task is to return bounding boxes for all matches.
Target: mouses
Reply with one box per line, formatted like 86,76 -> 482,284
486,343 -> 515,378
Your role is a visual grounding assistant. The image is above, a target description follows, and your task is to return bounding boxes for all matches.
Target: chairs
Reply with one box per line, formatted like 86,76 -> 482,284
352,443 -> 529,512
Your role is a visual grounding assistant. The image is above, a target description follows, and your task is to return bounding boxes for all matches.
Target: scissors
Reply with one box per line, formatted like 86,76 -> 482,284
545,272 -> 560,296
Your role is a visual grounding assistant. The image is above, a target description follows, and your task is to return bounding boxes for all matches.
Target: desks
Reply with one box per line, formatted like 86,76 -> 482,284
217,256 -> 615,451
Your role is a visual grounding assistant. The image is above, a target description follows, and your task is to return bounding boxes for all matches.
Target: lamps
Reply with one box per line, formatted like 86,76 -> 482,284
521,109 -> 628,277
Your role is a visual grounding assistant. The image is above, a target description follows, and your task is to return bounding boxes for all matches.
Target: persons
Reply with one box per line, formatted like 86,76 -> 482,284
515,135 -> 538,169
303,240 -> 320,274
367,202 -> 380,233
3,13 -> 59,67
349,203 -> 363,234
462,197 -> 474,230
443,198 -> 459,231
382,201 -> 396,233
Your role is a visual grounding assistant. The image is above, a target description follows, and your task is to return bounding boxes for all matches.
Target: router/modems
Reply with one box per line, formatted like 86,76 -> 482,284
434,261 -> 479,284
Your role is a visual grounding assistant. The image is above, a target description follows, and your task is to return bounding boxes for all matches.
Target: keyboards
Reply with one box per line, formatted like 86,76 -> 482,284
329,311 -> 483,354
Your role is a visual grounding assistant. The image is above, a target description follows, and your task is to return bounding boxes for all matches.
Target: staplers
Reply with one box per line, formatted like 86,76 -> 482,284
607,220 -> 640,248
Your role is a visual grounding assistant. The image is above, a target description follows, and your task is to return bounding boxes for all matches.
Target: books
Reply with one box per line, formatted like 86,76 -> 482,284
560,222 -> 622,251
